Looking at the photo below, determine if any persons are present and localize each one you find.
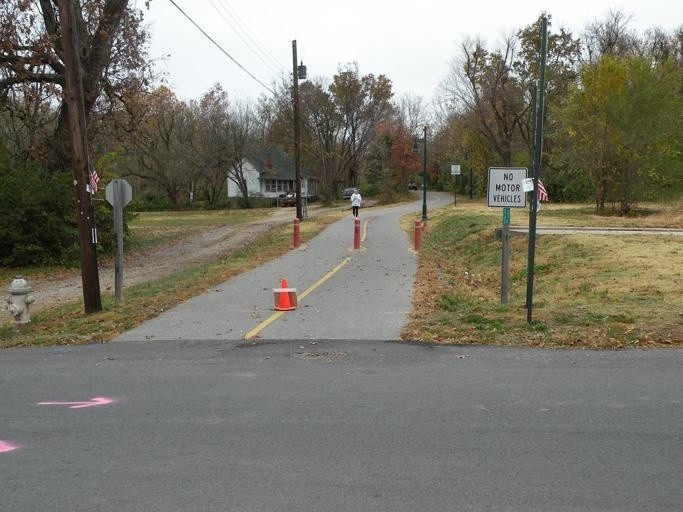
[350,189,361,219]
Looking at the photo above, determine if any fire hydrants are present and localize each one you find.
[5,274,35,329]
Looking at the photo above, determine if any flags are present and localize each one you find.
[87,157,100,194]
[537,176,549,202]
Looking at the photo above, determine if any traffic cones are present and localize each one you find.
[273,278,297,312]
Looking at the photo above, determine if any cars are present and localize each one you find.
[340,185,362,201]
[407,182,417,190]
[275,190,308,207]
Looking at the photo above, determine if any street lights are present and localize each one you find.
[290,57,307,222]
[431,160,440,192]
[410,124,430,222]
